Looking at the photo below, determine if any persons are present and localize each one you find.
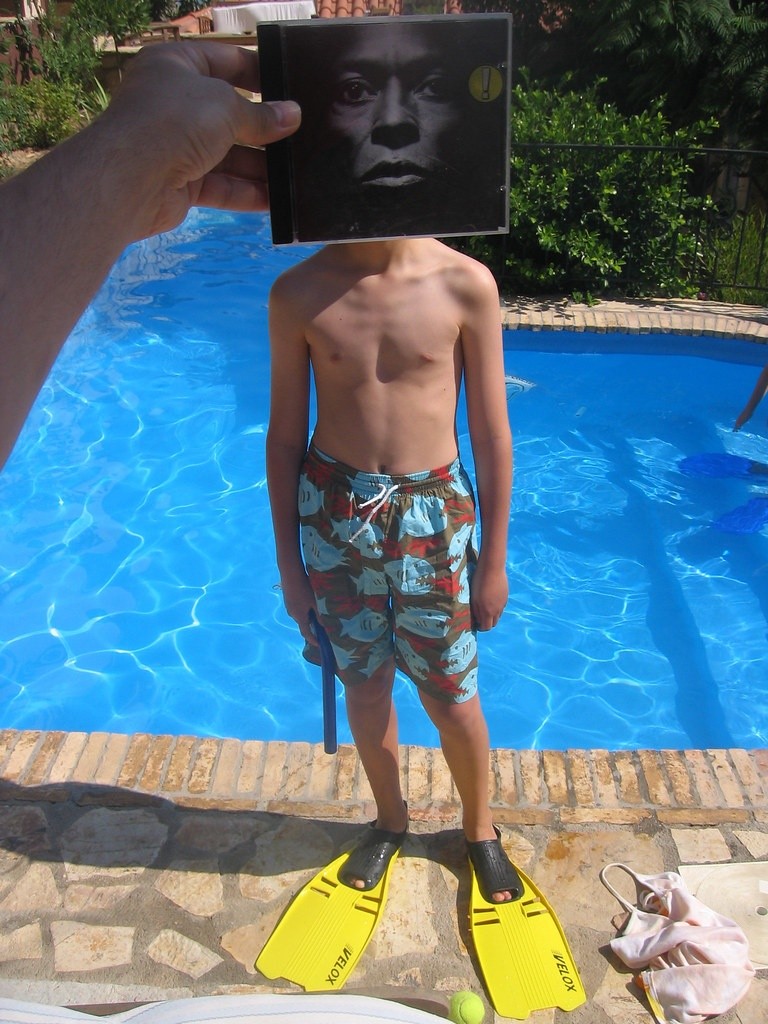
[263,236,525,905]
[309,22,501,236]
[0,33,300,471]
